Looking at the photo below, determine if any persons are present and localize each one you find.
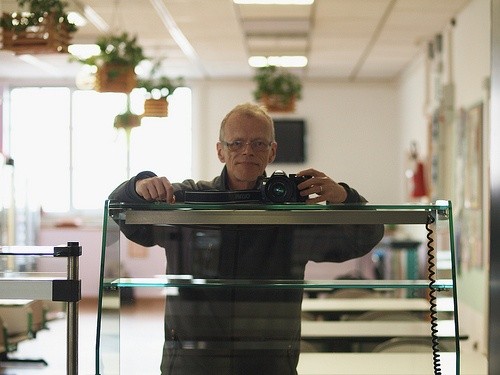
[105,103,385,375]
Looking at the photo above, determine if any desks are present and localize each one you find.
[297,279,456,375]
[0,298,48,366]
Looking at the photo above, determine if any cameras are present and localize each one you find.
[255,170,312,203]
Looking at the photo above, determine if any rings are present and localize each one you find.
[319,184,325,195]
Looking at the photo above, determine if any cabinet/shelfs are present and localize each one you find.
[95,197,461,375]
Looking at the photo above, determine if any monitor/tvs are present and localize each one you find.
[272,119,305,163]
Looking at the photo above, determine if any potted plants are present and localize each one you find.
[144,61,181,116]
[0,0,76,57]
[79,32,148,93]
[254,65,304,112]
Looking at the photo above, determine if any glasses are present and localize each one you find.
[221,139,274,150]
